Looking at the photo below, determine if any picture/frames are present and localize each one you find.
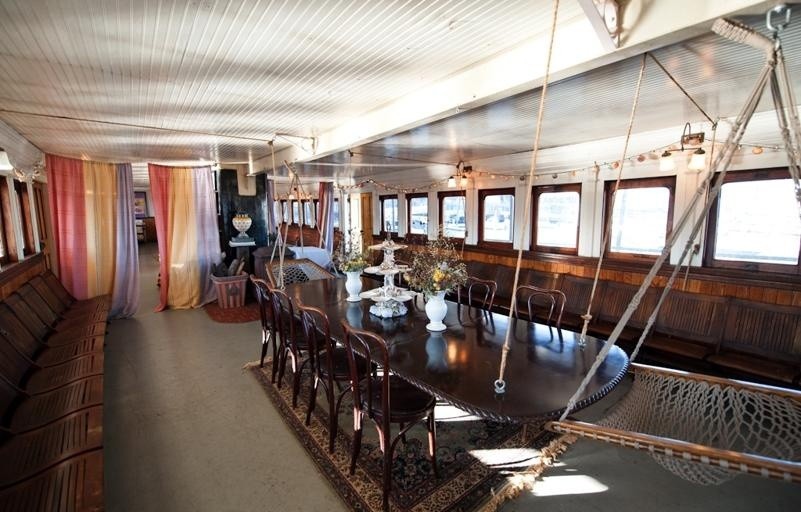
[133,191,147,219]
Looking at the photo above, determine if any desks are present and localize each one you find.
[270,275,629,445]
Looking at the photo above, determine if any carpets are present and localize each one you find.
[203,297,271,324]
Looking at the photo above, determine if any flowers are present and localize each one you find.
[333,229,370,273]
[408,230,467,293]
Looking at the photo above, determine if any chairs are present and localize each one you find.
[336,317,438,512]
[1,270,113,512]
[294,301,377,453]
[250,276,299,383]
[269,290,336,409]
[469,261,799,386]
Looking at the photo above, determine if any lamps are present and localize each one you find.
[0,145,24,182]
[448,160,468,187]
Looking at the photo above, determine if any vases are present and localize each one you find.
[231,215,251,238]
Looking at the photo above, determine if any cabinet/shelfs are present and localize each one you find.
[359,222,416,317]
